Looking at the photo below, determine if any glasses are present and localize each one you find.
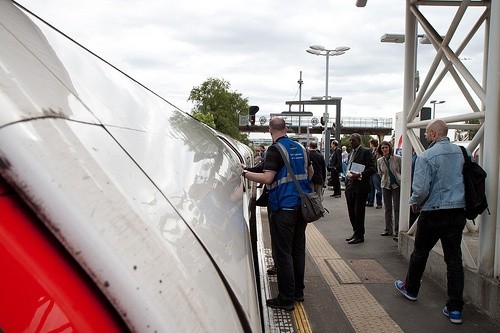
[423,129,432,136]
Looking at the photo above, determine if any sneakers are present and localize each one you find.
[394,279,417,301]
[442,304,463,324]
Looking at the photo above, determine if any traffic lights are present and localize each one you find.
[249,106,259,116]
[249,115,256,123]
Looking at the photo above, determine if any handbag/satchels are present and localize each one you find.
[298,192,325,223]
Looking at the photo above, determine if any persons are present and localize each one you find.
[409,138,421,229]
[308,141,326,202]
[254,149,261,166]
[256,145,267,188]
[394,119,475,323]
[327,140,349,198]
[242,119,314,310]
[301,141,308,153]
[339,133,401,244]
[242,142,276,276]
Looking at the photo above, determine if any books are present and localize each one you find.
[350,162,365,180]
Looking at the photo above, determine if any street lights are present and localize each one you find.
[306,44,350,118]
[430,100,446,119]
[379,33,447,101]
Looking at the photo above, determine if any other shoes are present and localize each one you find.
[267,266,277,274]
[266,296,295,311]
[394,232,398,236]
[381,231,392,236]
[330,194,341,198]
[346,231,364,244]
[276,293,304,302]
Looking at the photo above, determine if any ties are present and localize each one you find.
[349,150,355,163]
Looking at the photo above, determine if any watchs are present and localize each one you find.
[243,170,248,176]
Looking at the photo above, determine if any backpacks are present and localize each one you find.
[458,145,488,220]
